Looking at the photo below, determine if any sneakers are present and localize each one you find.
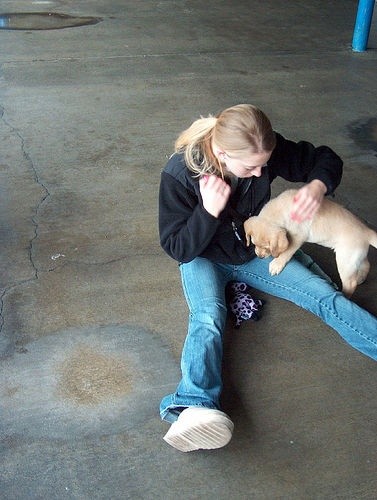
[163,405,236,454]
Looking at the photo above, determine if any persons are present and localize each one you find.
[156,102,377,453]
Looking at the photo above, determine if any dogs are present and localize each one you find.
[243,188,377,300]
